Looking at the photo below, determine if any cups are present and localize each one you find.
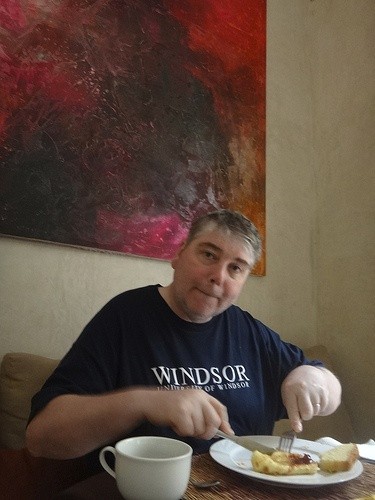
[99,436,193,500]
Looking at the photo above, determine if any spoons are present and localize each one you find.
[189,478,221,489]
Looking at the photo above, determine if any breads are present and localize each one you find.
[251,450,316,475]
[318,442,359,472]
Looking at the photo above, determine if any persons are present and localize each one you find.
[25,208,343,475]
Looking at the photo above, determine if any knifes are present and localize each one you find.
[215,429,277,456]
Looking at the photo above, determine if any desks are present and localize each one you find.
[55,443,375,500]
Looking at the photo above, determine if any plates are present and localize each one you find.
[209,436,364,489]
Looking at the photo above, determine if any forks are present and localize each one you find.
[278,415,303,454]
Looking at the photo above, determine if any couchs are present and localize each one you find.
[0,344,359,500]
